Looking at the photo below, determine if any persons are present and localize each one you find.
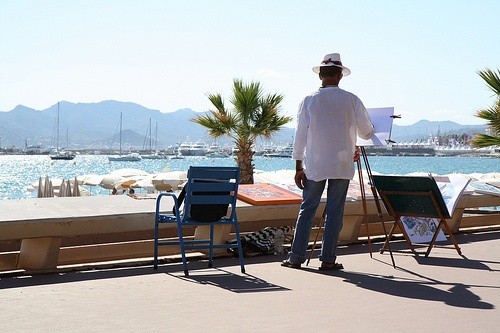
[281,52,373,271]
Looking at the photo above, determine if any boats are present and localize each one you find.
[139,116,294,160]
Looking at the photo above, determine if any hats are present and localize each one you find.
[312,53,352,78]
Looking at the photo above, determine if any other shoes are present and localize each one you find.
[281,259,301,268]
[319,263,343,271]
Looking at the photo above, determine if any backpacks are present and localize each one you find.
[173,179,230,222]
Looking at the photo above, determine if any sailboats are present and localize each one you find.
[107,111,143,162]
[22,101,77,161]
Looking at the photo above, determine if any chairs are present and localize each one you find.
[152,166,244,275]
